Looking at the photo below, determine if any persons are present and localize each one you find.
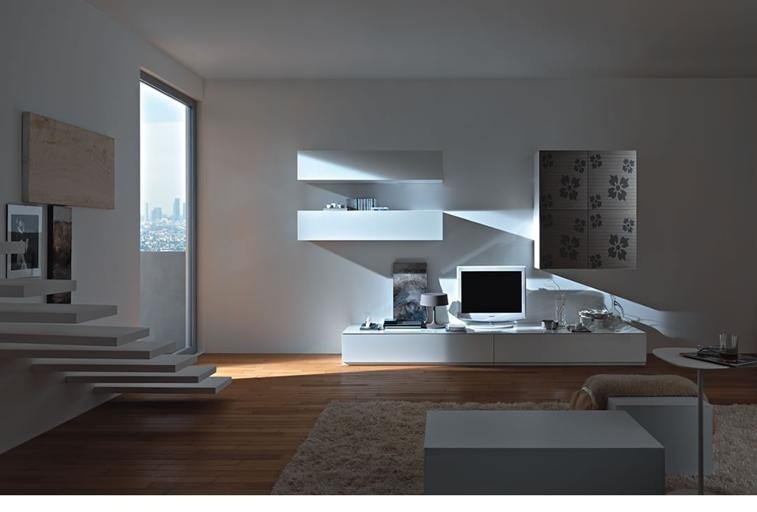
[15,215,32,269]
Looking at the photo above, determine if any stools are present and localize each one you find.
[605,396,712,476]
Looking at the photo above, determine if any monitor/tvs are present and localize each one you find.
[457,266,526,329]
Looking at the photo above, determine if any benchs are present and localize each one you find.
[424,411,666,496]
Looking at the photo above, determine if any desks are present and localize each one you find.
[652,347,757,492]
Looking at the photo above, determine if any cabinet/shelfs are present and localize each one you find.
[534,150,638,271]
[340,322,648,367]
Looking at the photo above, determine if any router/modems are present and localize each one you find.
[445,323,465,331]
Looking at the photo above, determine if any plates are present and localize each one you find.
[586,310,613,319]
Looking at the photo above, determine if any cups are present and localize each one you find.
[574,322,582,330]
[720,332,738,361]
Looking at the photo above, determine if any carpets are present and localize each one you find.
[270,399,757,495]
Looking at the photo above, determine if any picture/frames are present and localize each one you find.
[5,202,72,304]
